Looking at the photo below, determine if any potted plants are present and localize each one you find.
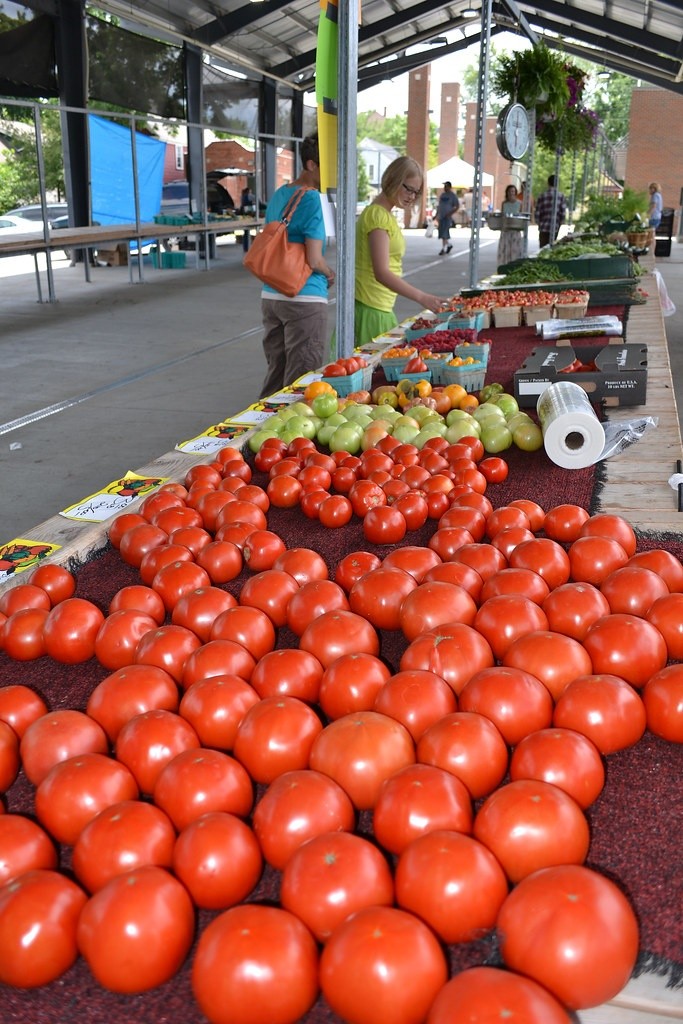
[626,226,653,249]
[498,38,571,103]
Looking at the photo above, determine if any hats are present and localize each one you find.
[443,182,451,187]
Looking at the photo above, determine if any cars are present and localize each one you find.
[0,203,71,259]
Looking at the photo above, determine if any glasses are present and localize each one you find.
[403,184,421,197]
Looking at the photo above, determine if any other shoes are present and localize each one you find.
[447,245,452,252]
[439,250,446,255]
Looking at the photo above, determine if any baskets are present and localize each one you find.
[321,309,492,400]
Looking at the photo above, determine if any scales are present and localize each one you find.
[486,71,530,229]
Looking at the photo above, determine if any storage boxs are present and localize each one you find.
[514,341,647,409]
[320,291,591,396]
[95,243,128,266]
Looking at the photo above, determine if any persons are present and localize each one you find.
[259,132,337,400]
[241,187,256,206]
[451,188,491,228]
[518,181,535,225]
[328,155,453,363]
[534,175,569,248]
[498,184,527,267]
[431,182,460,255]
[647,182,663,257]
[425,155,495,211]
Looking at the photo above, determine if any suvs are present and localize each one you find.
[164,165,253,245]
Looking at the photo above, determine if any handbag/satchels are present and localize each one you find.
[426,220,434,237]
[243,187,316,297]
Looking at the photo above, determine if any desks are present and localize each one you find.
[0,219,264,302]
[0,304,683,1024]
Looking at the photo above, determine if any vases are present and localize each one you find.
[541,110,558,123]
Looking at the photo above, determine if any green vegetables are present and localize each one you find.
[537,239,647,278]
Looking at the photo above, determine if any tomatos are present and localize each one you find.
[0,290,683,1024]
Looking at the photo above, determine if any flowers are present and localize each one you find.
[535,64,601,155]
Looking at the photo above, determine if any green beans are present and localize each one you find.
[495,260,575,286]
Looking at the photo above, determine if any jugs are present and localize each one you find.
[603,213,641,234]
